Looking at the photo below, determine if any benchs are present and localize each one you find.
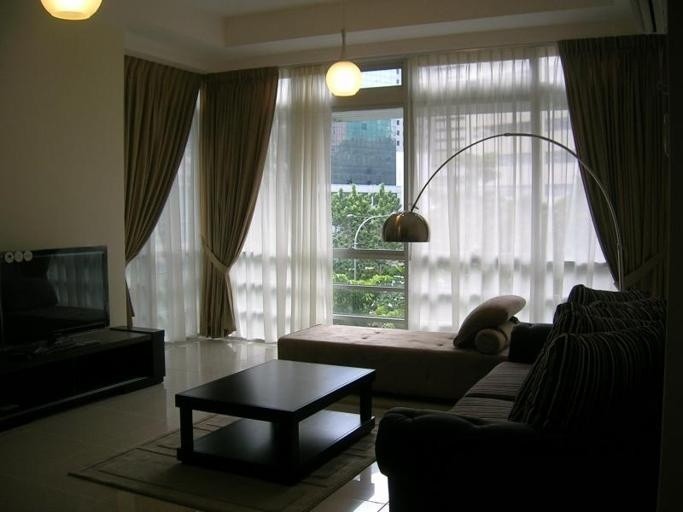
[278,321,520,400]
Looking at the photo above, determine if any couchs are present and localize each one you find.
[375,322,664,512]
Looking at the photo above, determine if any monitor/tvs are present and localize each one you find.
[0,247,109,355]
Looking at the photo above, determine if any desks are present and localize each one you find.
[0,325,165,432]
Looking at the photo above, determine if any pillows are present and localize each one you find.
[453,295,526,349]
[475,316,520,354]
[506,286,665,429]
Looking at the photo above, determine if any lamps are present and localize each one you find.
[381,133,624,292]
[325,29,361,97]
[41,0,102,20]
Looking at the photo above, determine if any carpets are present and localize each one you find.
[67,404,380,512]
